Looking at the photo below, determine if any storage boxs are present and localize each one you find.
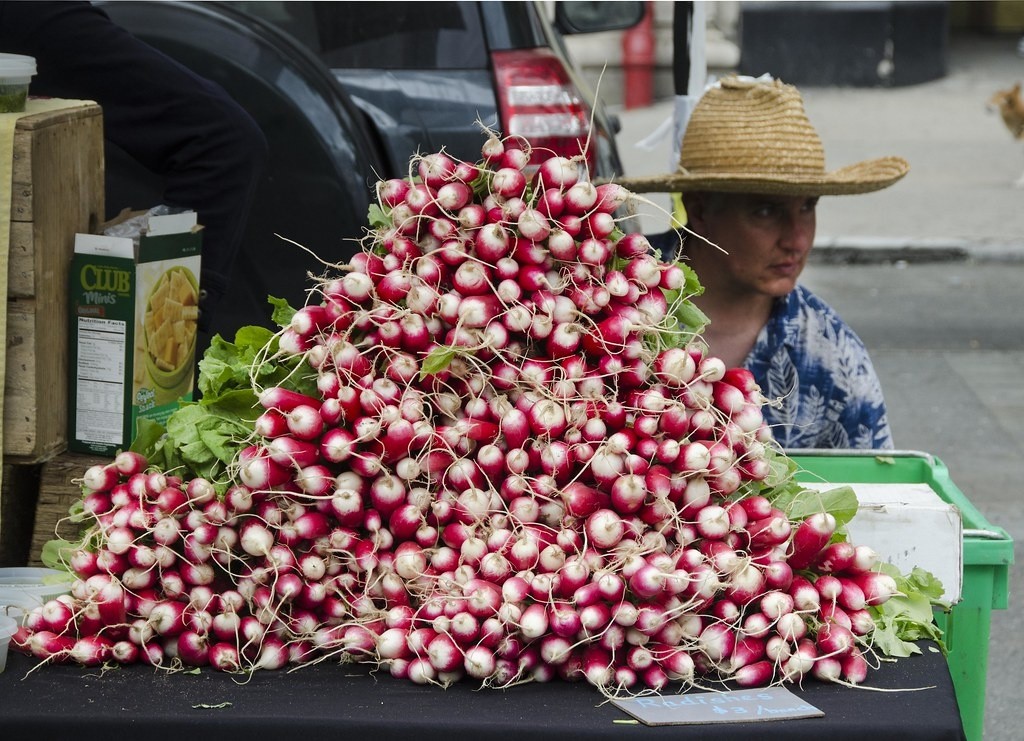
[1,97,106,466]
[798,481,965,607]
[781,449,1014,741]
[68,208,205,459]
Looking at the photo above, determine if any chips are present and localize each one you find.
[144,268,198,371]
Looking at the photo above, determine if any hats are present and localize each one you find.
[598,75,911,197]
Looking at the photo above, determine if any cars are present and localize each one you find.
[0,0,640,339]
[541,2,710,235]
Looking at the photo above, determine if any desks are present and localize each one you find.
[0,620,967,741]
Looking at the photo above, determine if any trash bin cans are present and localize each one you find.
[766,445,1015,741]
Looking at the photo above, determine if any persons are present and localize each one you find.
[643,101,895,450]
